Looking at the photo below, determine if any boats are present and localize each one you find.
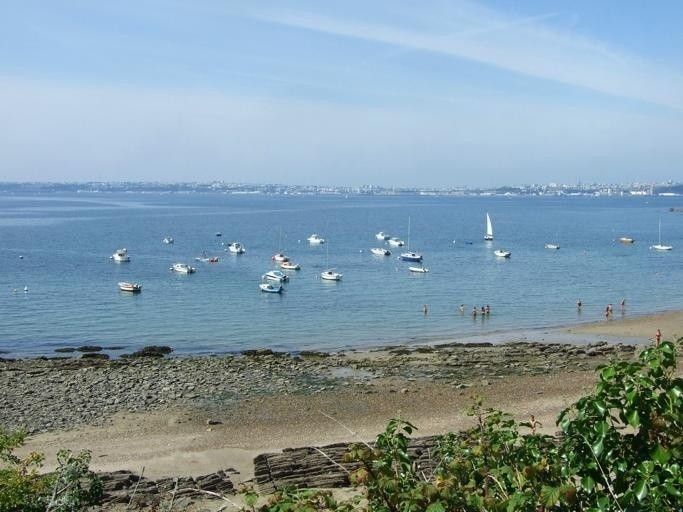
[493,249,511,257]
[110,249,131,263]
[162,232,247,273]
[650,220,673,250]
[259,233,343,293]
[371,217,430,273]
[117,281,143,291]
[544,243,560,249]
[619,237,635,244]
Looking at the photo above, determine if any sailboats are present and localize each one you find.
[482,212,494,240]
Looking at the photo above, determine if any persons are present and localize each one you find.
[470,305,477,317]
[575,296,581,312]
[605,306,610,321]
[655,327,661,346]
[608,303,612,316]
[458,303,464,313]
[480,306,485,316]
[620,296,625,312]
[485,304,490,314]
[422,304,428,314]
